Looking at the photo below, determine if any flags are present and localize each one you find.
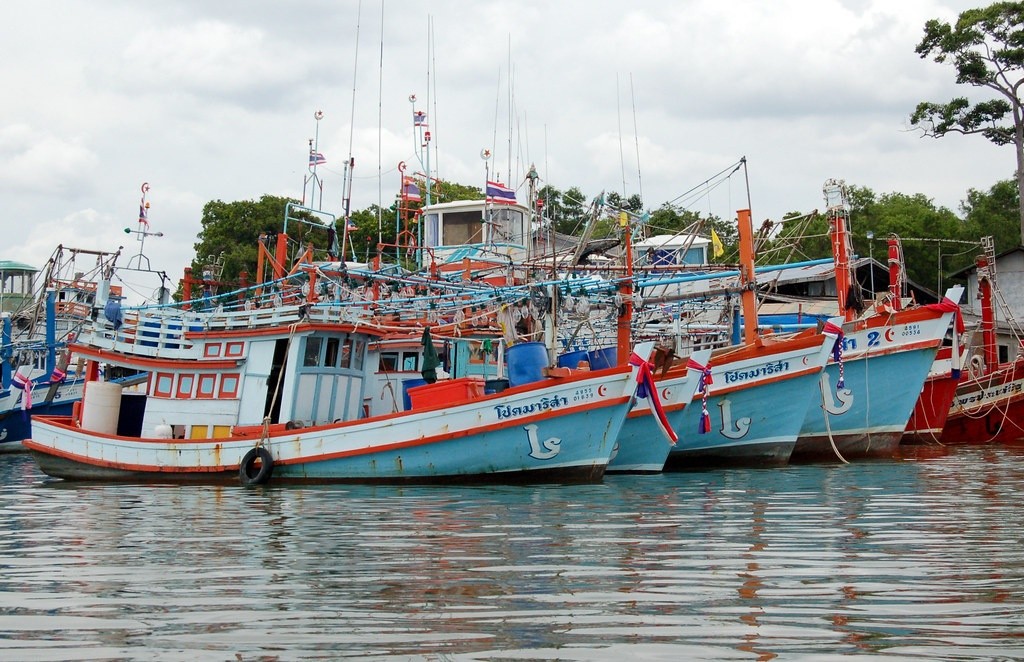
[712,229,724,259]
[485,181,518,205]
[403,176,421,198]
[138,203,149,232]
[347,218,358,231]
[309,153,326,165]
[414,111,431,127]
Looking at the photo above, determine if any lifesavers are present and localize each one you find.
[239,447,274,485]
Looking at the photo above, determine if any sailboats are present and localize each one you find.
[0,0,1023,484]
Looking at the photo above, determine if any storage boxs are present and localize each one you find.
[406,377,485,409]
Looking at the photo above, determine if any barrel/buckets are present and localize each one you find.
[558,350,588,368]
[589,347,617,370]
[82,381,122,435]
[401,378,427,410]
[506,342,550,388]
